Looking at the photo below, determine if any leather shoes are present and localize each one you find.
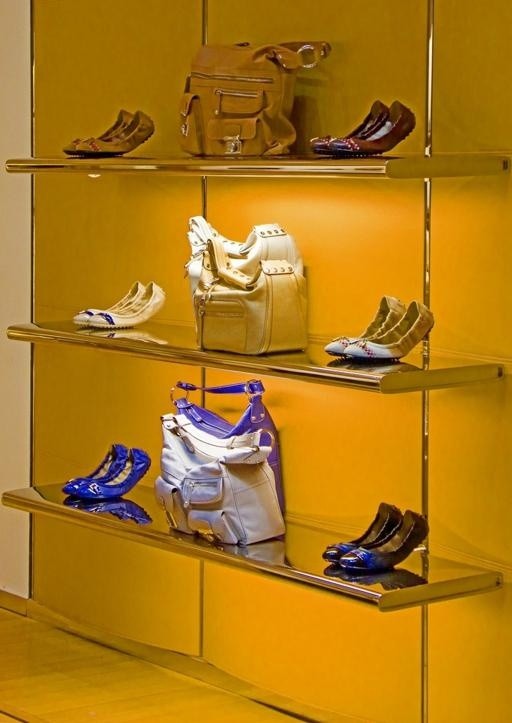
[309,100,415,153]
[63,444,150,499]
[325,564,428,589]
[324,296,434,358]
[323,503,428,570]
[63,496,152,525]
[73,281,165,329]
[63,110,154,153]
[77,330,167,344]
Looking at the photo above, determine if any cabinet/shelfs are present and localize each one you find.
[0,0,512,722]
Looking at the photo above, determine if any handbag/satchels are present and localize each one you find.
[154,379,287,545]
[184,216,307,356]
[178,42,331,157]
